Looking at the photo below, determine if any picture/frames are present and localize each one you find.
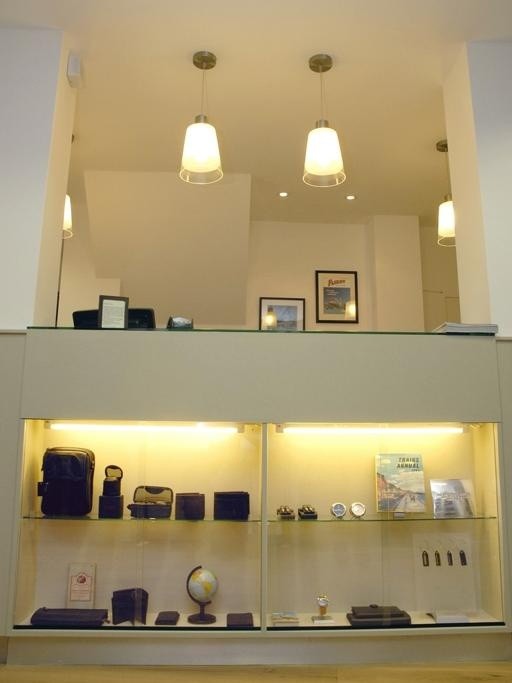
[315,269,360,324]
[98,295,130,329]
[258,297,306,331]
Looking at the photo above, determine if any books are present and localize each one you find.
[431,478,477,518]
[375,454,427,513]
[433,321,499,334]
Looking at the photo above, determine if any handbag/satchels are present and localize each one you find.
[37,447,95,516]
[347,604,411,627]
[31,608,107,626]
[128,486,173,518]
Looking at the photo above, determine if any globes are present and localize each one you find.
[185,565,218,624]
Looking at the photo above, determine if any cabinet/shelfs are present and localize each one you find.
[260,419,512,638]
[12,417,266,631]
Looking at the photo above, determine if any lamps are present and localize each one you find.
[275,424,472,438]
[60,195,75,239]
[303,53,347,188]
[435,139,458,249]
[43,421,246,436]
[179,50,225,186]
